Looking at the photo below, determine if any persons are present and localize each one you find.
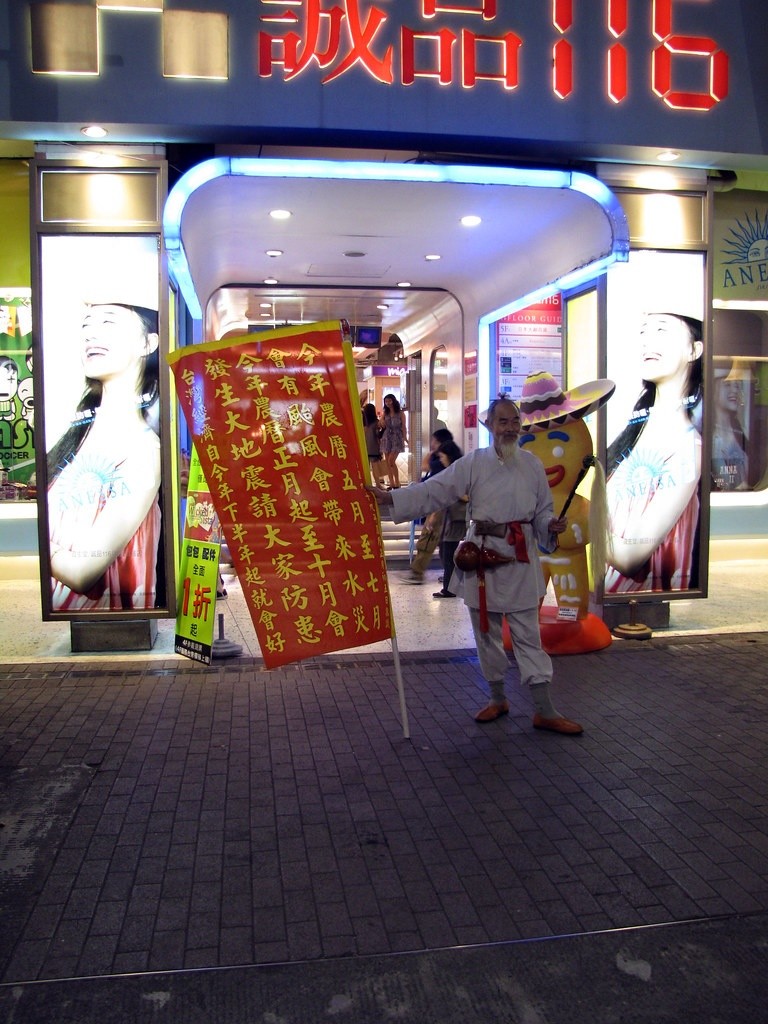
[44,293,164,610]
[365,394,585,733]
[399,429,469,597]
[711,369,750,491]
[362,394,407,490]
[606,302,701,593]
[477,369,615,620]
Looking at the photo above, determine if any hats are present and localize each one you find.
[84,254,158,312]
[647,282,705,321]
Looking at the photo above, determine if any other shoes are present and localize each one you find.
[438,577,444,583]
[433,590,456,598]
[376,484,400,491]
[474,699,510,723]
[400,570,425,584]
[534,712,584,734]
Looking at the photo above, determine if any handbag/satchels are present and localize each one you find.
[449,501,467,523]
